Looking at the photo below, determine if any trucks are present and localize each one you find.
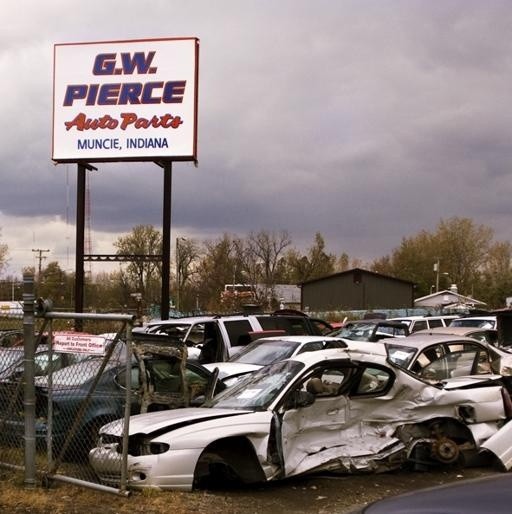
[221,282,262,309]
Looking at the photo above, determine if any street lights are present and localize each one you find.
[175,235,186,309]
[429,284,435,295]
[436,259,449,292]
